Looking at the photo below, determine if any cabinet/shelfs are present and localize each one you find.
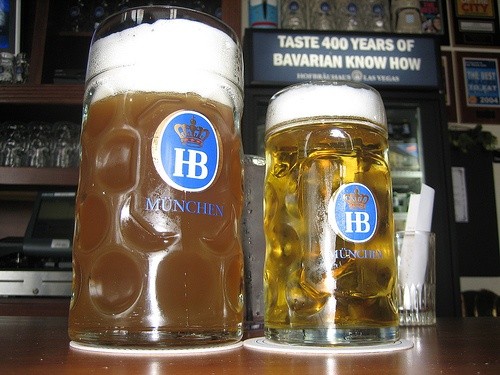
[0,0,242,183]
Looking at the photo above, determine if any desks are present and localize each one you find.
[0,297,500,375]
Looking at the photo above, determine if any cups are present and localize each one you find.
[67,4,247,347]
[0,119,81,169]
[249,0,442,32]
[262,78,401,348]
[0,51,30,86]
[393,229,438,327]
[246,156,266,329]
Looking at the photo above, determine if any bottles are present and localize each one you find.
[388,115,413,138]
[393,190,415,212]
